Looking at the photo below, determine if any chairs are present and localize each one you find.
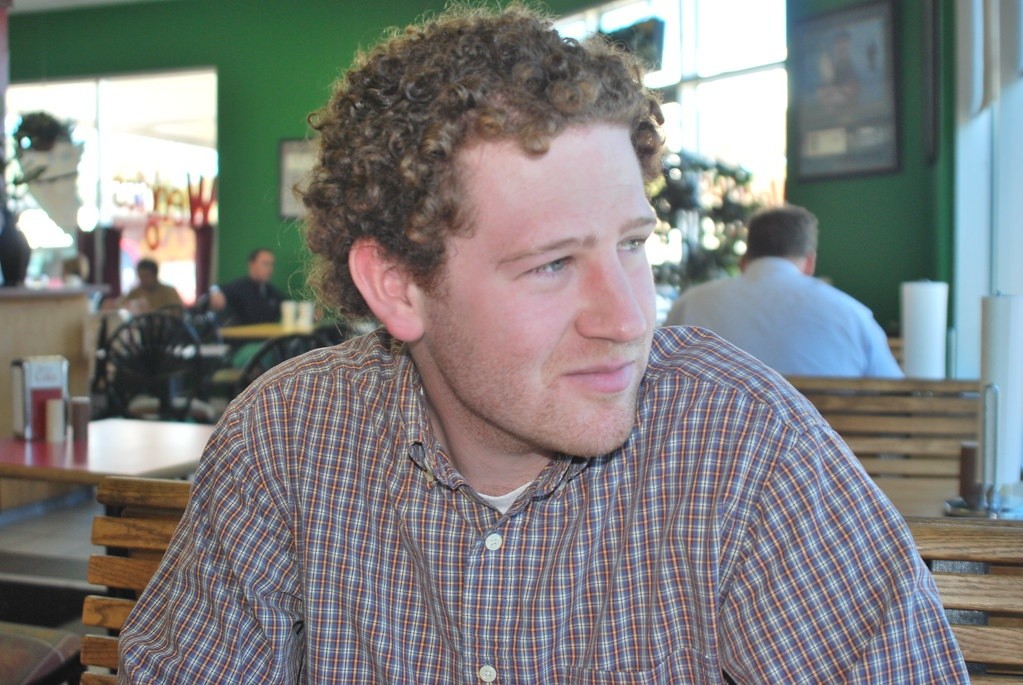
[92,294,359,426]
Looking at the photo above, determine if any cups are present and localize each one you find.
[297,302,312,328]
[281,302,296,328]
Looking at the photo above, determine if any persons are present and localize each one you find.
[206,248,300,369]
[112,258,184,418]
[115,0,970,685]
[664,206,906,378]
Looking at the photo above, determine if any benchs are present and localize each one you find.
[78,377,1023,685]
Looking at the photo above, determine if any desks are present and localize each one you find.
[218,321,317,340]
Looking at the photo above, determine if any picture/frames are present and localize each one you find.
[278,137,318,220]
[795,0,903,183]
